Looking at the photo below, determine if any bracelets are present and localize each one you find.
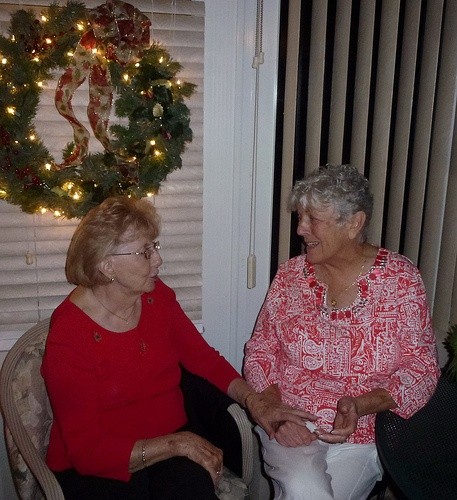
[142,439,148,468]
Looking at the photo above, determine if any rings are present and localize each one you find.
[217,471,220,475]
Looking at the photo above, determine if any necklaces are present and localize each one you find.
[91,283,137,324]
[320,241,366,306]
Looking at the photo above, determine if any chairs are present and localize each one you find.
[0,319,253,500]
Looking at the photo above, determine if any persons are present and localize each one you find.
[241,164,441,500]
[43,193,319,500]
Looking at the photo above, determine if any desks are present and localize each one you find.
[373,371,457,500]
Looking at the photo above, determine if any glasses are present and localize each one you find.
[102,239,161,258]
